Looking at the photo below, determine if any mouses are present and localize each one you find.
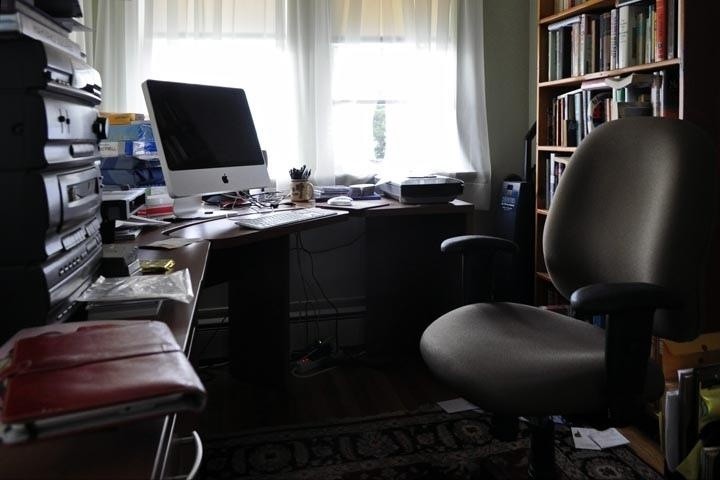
[326,195,353,206]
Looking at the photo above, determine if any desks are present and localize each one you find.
[0,189,476,480]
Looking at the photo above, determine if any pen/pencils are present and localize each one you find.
[289,165,311,179]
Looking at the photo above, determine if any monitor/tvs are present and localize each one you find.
[141,79,273,222]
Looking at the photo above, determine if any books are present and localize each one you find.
[545,152,574,210]
[546,67,678,147]
[544,1,678,81]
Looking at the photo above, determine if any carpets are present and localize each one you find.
[198,407,666,480]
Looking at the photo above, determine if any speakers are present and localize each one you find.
[494,179,527,290]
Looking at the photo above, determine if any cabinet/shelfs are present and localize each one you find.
[535,0,720,480]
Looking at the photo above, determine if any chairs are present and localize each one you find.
[419,115,717,480]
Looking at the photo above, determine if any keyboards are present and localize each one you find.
[235,207,337,231]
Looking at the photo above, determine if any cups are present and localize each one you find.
[289,178,315,202]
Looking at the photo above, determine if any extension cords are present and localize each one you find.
[296,345,338,374]
